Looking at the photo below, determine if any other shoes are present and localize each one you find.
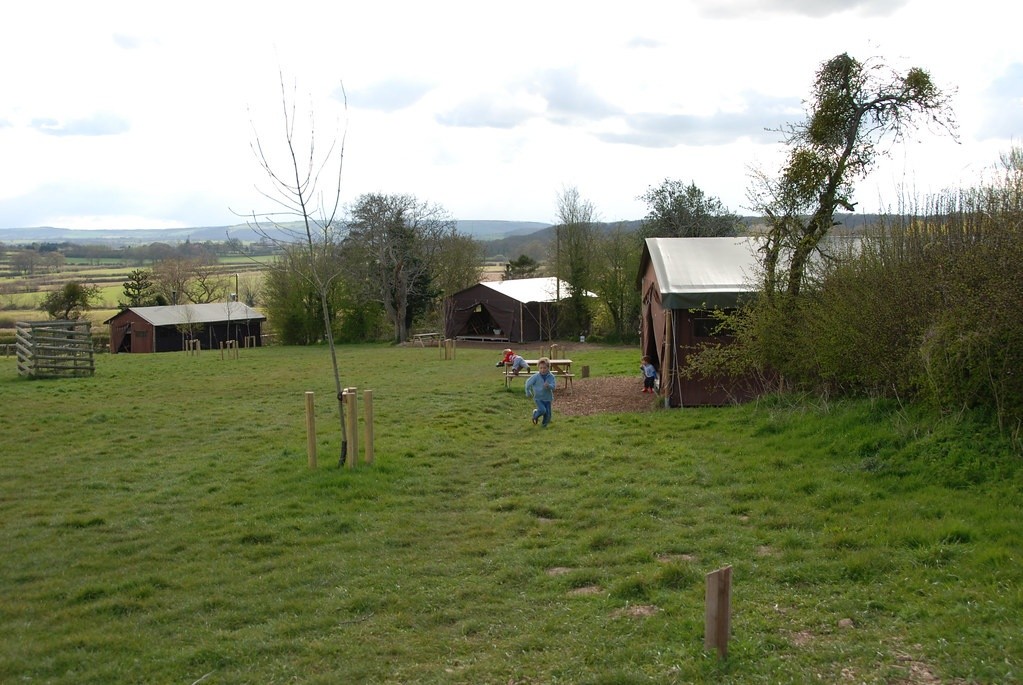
[641,387,648,392]
[513,370,518,375]
[528,367,530,374]
[648,387,652,393]
[533,409,538,424]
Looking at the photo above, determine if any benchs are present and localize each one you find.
[502,371,575,378]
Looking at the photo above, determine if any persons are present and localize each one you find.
[639,355,657,394]
[498,349,531,376]
[525,357,556,429]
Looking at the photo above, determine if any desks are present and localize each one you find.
[411,332,440,348]
[506,359,575,393]
